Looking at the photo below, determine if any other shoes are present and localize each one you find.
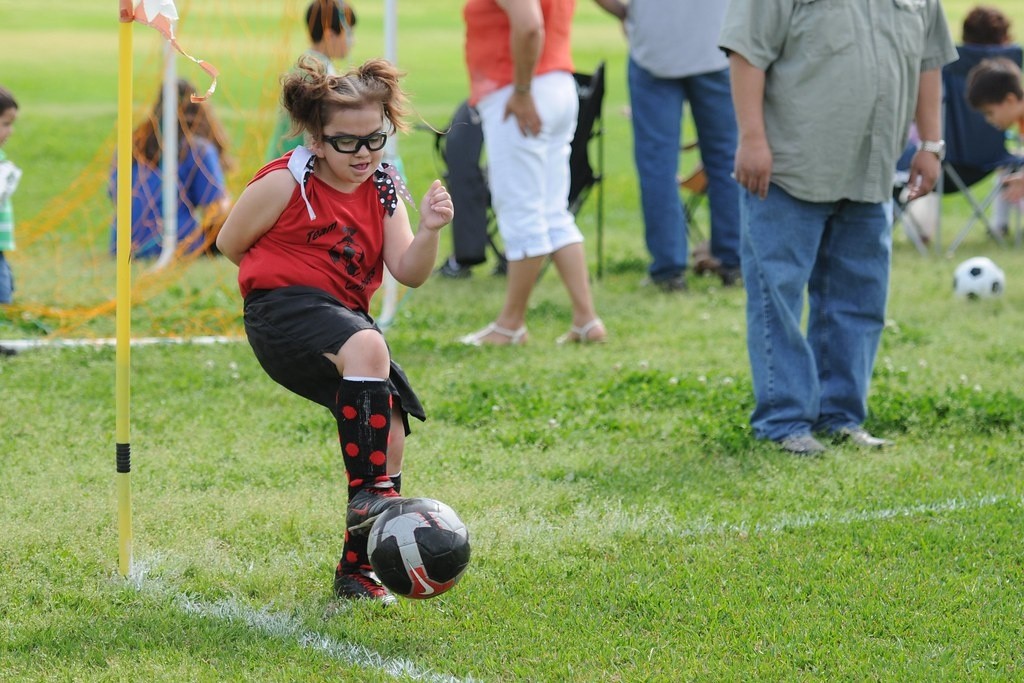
[719,266,744,289]
[488,258,508,277]
[638,273,689,298]
[431,259,471,280]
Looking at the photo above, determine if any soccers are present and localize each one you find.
[952,257,1009,302]
[366,496,472,600]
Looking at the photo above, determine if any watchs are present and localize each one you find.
[917,140,947,160]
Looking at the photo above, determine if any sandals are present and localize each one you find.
[461,323,527,349]
[556,319,606,347]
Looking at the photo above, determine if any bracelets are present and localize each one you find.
[514,85,529,91]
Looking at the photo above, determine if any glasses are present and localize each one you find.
[312,130,387,153]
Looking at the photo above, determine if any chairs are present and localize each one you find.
[886,43,1024,261]
[432,63,608,279]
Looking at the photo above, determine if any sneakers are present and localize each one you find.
[828,425,893,452]
[334,565,398,610]
[344,480,406,537]
[776,435,824,457]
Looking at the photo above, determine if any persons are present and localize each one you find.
[0,87,19,356]
[215,62,454,606]
[109,80,224,253]
[898,6,1024,243]
[597,1,742,289]
[436,1,605,347]
[718,0,959,452]
[266,0,355,162]
